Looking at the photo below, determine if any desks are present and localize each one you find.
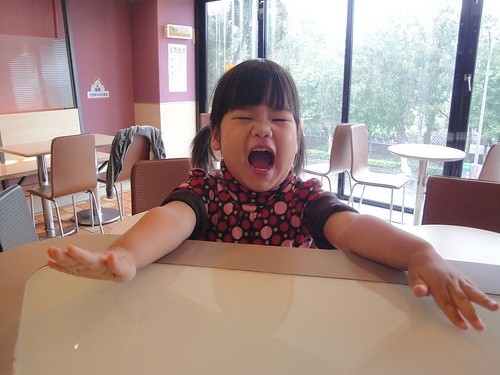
[0,133,122,240]
[0,234,500,375]
[388,143,466,225]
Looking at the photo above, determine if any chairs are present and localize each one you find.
[130,158,201,216]
[293,123,354,209]
[89,125,166,227]
[346,124,411,225]
[26,133,105,237]
[421,142,500,233]
[200,113,223,169]
[0,184,39,253]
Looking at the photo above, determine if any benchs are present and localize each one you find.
[0,151,111,190]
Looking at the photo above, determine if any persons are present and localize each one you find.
[46,57,500,330]
